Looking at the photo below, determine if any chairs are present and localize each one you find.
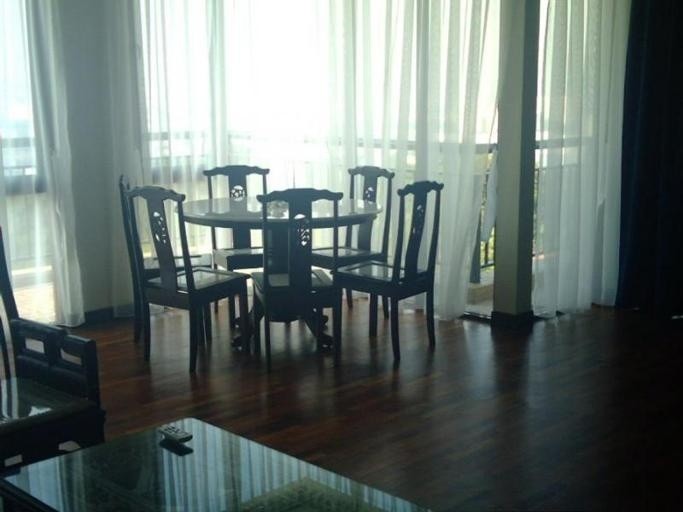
[310,166,395,318]
[329,179,444,365]
[1,312,108,475]
[205,165,269,312]
[114,174,212,348]
[252,188,343,372]
[124,186,251,373]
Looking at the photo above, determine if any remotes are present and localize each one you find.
[159,426,193,442]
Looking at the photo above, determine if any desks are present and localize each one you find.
[174,193,384,351]
